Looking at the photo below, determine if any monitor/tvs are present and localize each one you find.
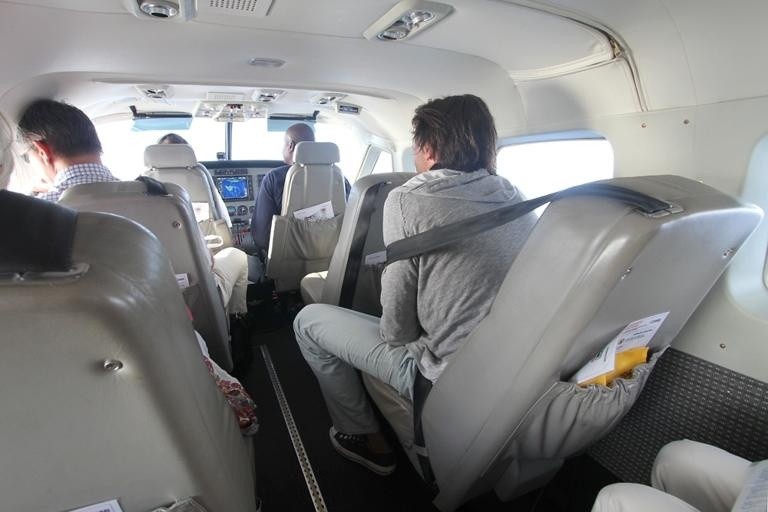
[213,175,254,201]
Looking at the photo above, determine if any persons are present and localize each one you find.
[0,109,264,436]
[291,88,546,479]
[160,129,237,231]
[15,96,257,333]
[242,122,351,281]
[592,432,767,510]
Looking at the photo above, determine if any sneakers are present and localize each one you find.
[329,425,400,477]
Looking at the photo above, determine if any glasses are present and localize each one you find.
[20,147,33,163]
